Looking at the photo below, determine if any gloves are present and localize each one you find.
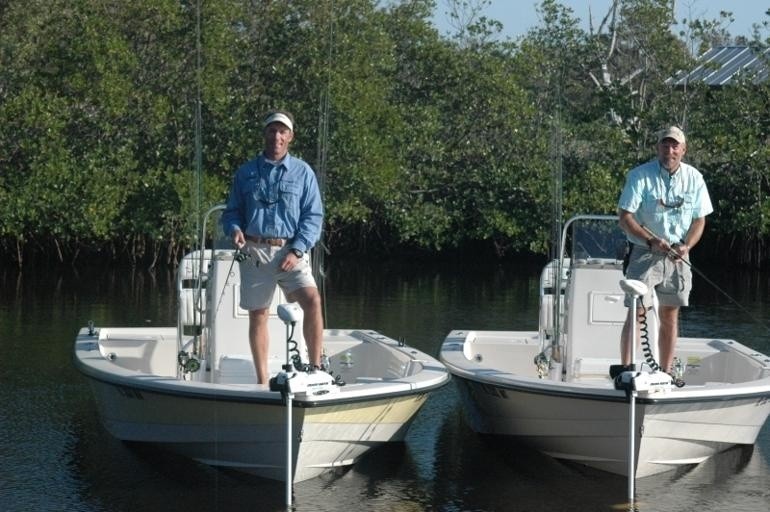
[647,235,670,252]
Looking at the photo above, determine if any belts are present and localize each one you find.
[244,235,283,246]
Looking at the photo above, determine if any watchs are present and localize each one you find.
[292,248,304,258]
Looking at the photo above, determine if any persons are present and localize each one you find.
[614,125,716,388]
[219,107,327,388]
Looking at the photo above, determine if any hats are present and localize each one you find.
[657,127,685,144]
[263,111,294,133]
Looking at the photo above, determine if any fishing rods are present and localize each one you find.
[208,240,245,338]
[640,223,770,332]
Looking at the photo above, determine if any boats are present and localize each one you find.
[437,71,770,503]
[69,1,455,491]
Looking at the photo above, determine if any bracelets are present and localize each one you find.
[646,236,653,246]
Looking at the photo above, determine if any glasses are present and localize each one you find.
[258,183,284,206]
[660,182,685,208]
